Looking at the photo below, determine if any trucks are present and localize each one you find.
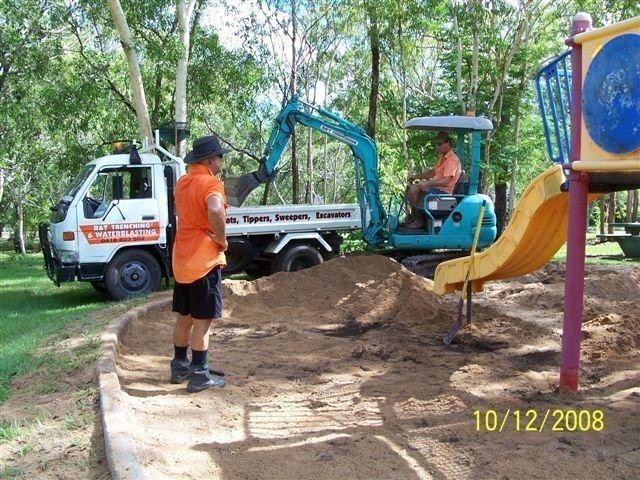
[38,129,370,301]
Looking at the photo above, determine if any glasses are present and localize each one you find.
[218,153,224,159]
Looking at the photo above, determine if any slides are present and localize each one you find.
[434,163,604,295]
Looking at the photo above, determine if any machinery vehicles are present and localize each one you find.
[223,93,498,278]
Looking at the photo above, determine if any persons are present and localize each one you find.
[169,135,229,394]
[397,131,462,230]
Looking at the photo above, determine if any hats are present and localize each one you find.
[182,136,231,163]
[430,132,451,143]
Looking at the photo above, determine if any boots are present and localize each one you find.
[185,367,226,392]
[169,360,191,383]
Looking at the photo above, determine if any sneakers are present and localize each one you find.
[406,219,425,229]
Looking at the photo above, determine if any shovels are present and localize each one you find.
[442,200,486,346]
